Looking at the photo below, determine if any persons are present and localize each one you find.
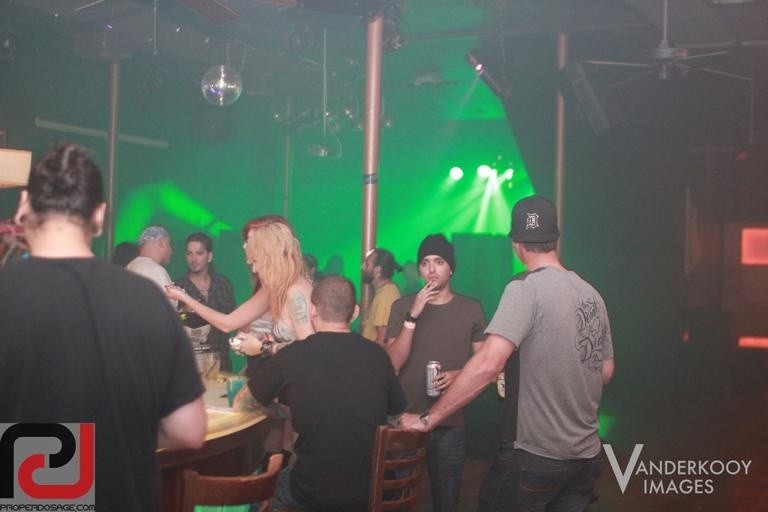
[1,137,210,511]
[227,272,408,511]
[122,223,180,315]
[379,233,491,512]
[174,233,238,373]
[357,247,407,353]
[162,216,294,375]
[399,194,615,511]
[226,223,316,407]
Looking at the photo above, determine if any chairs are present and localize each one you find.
[371,424,430,511]
[180,453,283,512]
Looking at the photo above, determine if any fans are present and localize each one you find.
[68,0,302,28]
[575,1,756,98]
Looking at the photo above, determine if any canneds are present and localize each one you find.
[426,360,442,398]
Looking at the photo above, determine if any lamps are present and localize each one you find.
[199,1,405,160]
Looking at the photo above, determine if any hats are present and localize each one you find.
[417,234,454,274]
[509,194,562,244]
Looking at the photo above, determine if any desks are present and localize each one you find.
[145,371,275,475]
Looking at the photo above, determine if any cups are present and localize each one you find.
[202,347,220,380]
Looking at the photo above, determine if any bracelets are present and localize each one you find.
[256,332,269,343]
[260,341,273,358]
[420,411,431,429]
[406,312,416,322]
[403,319,416,329]
